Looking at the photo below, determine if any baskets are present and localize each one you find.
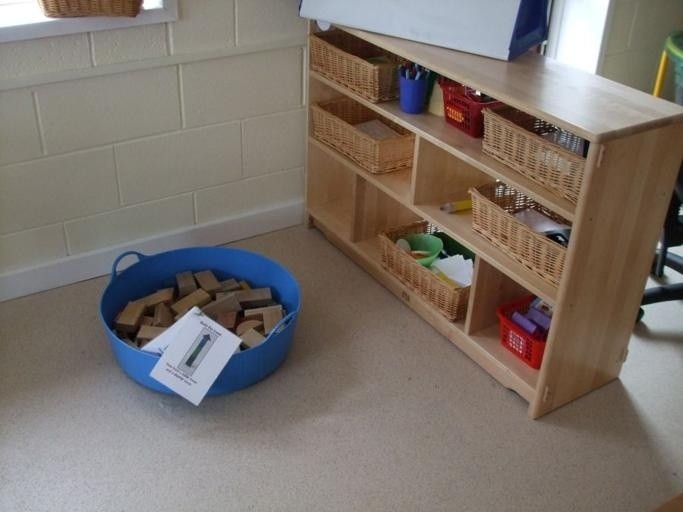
[311,96,415,173]
[470,182,572,288]
[38,0,143,17]
[481,106,587,207]
[497,295,546,370]
[309,30,415,103]
[379,221,471,321]
[440,76,536,137]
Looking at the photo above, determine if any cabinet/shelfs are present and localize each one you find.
[298,15,681,422]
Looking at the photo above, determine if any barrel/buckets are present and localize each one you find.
[398,74,430,113]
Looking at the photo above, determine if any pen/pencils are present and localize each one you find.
[440,199,473,213]
[398,60,430,80]
